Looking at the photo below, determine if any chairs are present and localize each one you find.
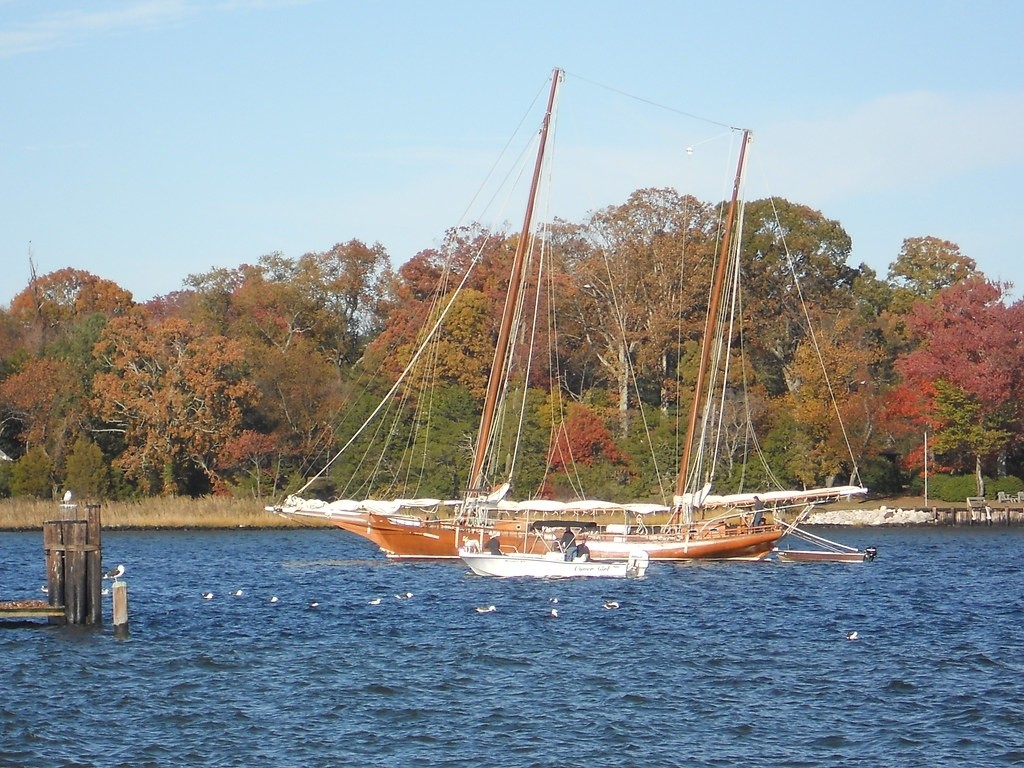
[998,491,1024,503]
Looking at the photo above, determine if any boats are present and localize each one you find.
[773,550,866,563]
[458,520,649,580]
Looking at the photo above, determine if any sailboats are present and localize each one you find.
[261,67,868,579]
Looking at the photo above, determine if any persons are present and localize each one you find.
[485,535,509,556]
[753,496,763,533]
[552,538,563,552]
[576,543,590,562]
[557,527,577,561]
[460,515,468,530]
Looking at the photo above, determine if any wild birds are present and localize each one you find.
[846,630,859,640]
[199,592,213,599]
[228,589,242,596]
[550,597,559,618]
[394,592,413,599]
[601,599,619,610]
[41,585,48,592]
[475,605,496,613]
[101,563,125,596]
[60,490,71,505]
[268,596,278,603]
[305,601,318,607]
[368,598,382,605]
